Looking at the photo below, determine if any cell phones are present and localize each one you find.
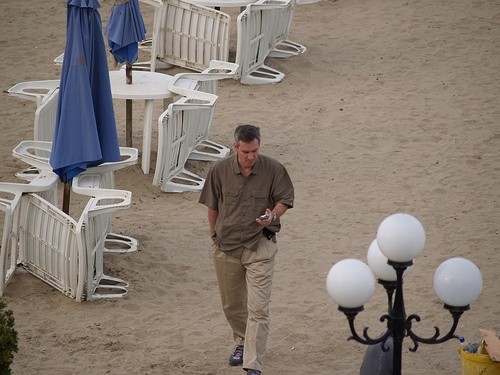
[259,215,269,221]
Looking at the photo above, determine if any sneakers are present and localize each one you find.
[228,344,244,366]
[247,369,262,375]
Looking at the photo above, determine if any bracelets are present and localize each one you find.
[272,210,277,222]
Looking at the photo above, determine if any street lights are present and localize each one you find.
[325,214,483,375]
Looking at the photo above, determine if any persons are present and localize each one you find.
[198,124,293,375]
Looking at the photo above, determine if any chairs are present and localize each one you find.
[0,0,322,304]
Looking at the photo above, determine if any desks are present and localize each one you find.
[109,68,176,147]
[187,0,258,11]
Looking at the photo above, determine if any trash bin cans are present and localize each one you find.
[457,328,500,375]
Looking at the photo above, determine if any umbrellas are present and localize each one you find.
[104,0,148,146]
[49,0,122,215]
